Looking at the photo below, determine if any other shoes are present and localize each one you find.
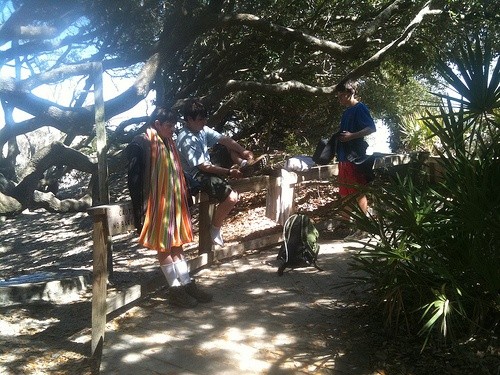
[240,153,270,176]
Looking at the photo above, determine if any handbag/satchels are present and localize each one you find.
[312,138,336,165]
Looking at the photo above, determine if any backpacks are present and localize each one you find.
[278,214,320,274]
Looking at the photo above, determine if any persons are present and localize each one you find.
[175,105,271,247]
[124,107,213,307]
[334,81,376,238]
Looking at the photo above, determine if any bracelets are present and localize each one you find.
[227,169,232,176]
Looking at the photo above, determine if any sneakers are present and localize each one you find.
[168,286,198,308]
[344,227,363,242]
[181,280,212,302]
[338,221,349,237]
